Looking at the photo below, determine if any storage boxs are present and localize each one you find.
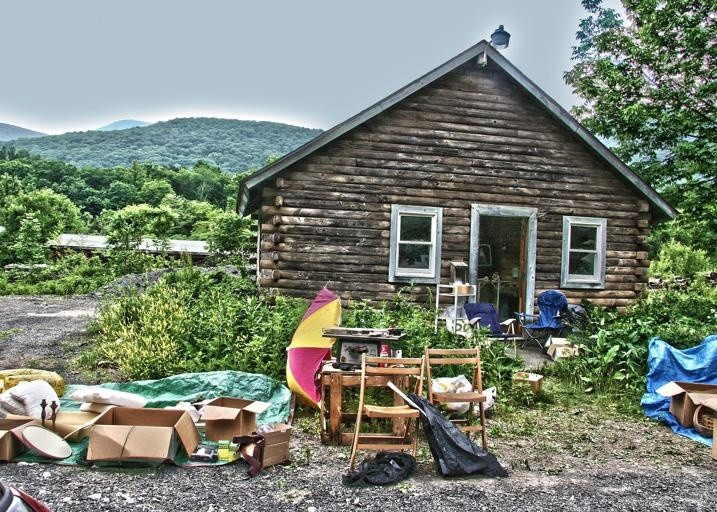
[190,395,272,444]
[655,380,717,429]
[61,406,201,465]
[513,372,543,393]
[0,417,42,462]
[544,336,572,348]
[256,422,292,469]
[701,397,717,461]
[547,344,577,362]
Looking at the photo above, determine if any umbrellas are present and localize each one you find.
[286,280,341,411]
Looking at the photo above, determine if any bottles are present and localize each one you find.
[380,345,388,367]
[391,348,402,359]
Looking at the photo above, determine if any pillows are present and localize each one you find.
[1,388,27,417]
[0,406,13,419]
[68,387,145,408]
[10,378,59,418]
[80,402,113,414]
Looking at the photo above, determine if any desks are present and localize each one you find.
[316,357,410,445]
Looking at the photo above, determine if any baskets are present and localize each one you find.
[693,405,714,438]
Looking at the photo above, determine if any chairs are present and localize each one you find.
[424,346,488,449]
[348,352,426,469]
[464,300,517,358]
[513,290,568,358]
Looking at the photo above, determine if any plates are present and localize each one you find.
[23,425,72,459]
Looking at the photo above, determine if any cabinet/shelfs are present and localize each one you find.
[435,284,478,335]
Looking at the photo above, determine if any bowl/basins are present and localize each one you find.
[458,286,472,294]
